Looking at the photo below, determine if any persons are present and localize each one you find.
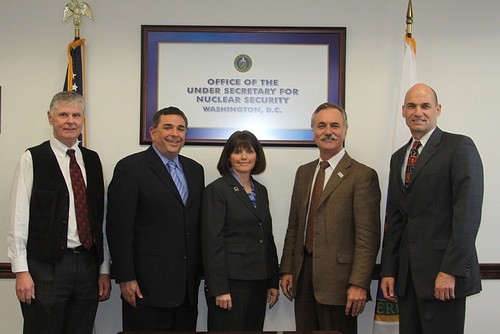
[380,83,483,334]
[201,130,279,334]
[277,103,382,334]
[107,107,205,334]
[9,91,111,334]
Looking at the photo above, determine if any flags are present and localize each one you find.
[373,36,420,334]
[64,36,88,147]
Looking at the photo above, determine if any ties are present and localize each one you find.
[66,148,93,251]
[168,161,188,206]
[404,140,422,188]
[305,160,330,255]
